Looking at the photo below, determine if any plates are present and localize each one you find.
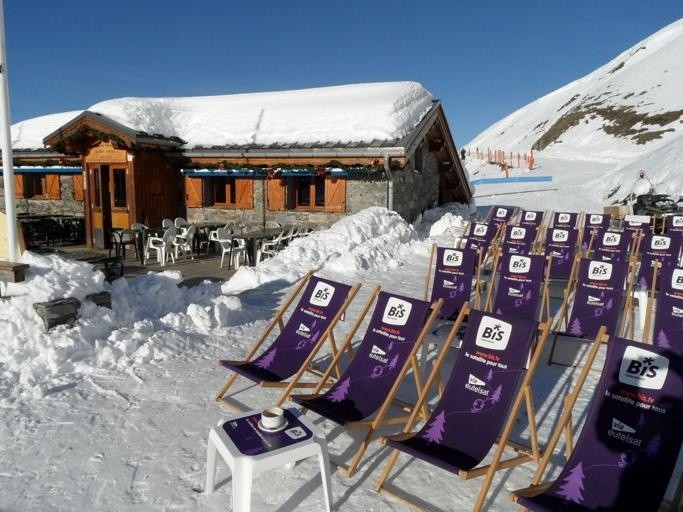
[257,417,289,432]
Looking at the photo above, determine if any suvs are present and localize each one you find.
[626,190,682,236]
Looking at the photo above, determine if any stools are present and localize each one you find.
[205,407,333,512]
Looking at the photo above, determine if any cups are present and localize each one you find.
[261,408,284,428]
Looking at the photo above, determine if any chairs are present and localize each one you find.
[106,217,309,282]
